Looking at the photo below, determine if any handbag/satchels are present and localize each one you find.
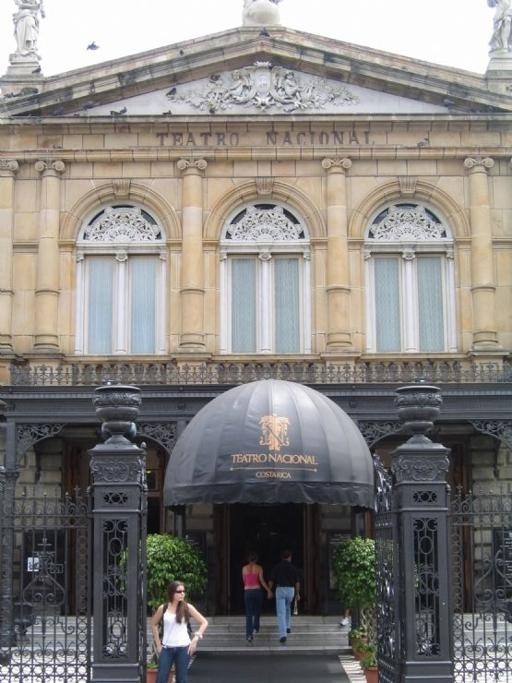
[292,594,301,616]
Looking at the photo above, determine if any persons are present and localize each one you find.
[338,606,351,627]
[149,579,209,683]
[240,550,274,643]
[266,549,302,644]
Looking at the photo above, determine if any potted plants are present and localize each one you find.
[118,533,208,683]
[332,534,417,681]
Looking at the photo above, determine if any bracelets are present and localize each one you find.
[190,631,204,640]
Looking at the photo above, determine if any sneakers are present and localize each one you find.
[340,617,349,626]
[278,626,292,644]
[246,636,254,647]
